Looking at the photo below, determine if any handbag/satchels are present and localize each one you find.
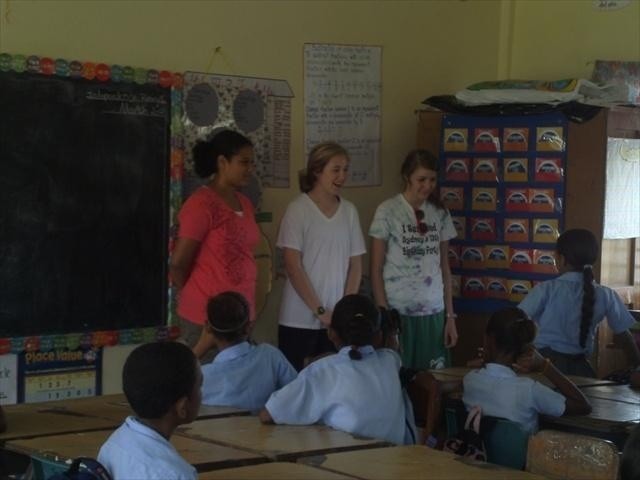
[443,406,487,462]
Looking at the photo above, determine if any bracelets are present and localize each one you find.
[445,313,457,319]
[537,357,552,376]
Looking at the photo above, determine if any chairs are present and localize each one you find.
[482,417,525,469]
[526,431,619,480]
[30,450,111,480]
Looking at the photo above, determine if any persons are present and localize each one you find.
[275,141,365,363]
[193,290,299,417]
[96,342,203,480]
[462,308,593,436]
[370,148,457,447]
[169,130,262,363]
[517,228,640,376]
[381,307,444,446]
[259,294,408,453]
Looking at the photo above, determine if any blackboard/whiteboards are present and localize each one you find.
[0,55,185,354]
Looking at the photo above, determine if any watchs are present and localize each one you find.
[313,306,328,316]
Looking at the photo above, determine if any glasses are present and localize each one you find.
[415,207,427,234]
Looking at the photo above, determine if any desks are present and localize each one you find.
[6,429,264,471]
[297,444,544,480]
[198,462,358,480]
[540,385,640,451]
[518,371,617,390]
[174,415,390,461]
[416,366,478,445]
[38,394,251,422]
[0,402,122,440]
